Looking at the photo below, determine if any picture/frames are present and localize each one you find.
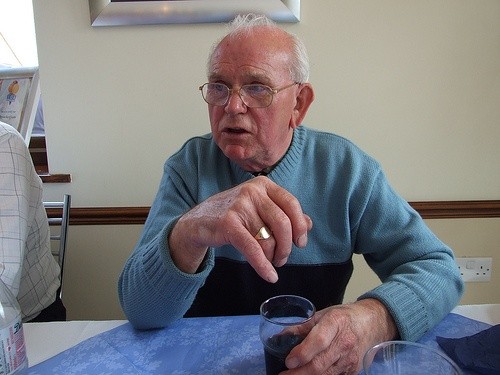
[0,65,41,149]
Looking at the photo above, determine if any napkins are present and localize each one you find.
[436,324,500,375]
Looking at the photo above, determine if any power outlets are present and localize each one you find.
[454,257,492,282]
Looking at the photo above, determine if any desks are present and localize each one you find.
[19,304,500,375]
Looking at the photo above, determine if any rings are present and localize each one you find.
[254,226,272,239]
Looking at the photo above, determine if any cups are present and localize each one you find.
[363,340,465,375]
[259,295,316,375]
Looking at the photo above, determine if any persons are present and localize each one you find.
[0,121,67,323]
[117,13,464,375]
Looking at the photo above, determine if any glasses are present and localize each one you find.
[199,81,301,108]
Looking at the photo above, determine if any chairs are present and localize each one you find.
[43,195,71,300]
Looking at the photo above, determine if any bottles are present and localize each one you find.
[0,279,29,375]
